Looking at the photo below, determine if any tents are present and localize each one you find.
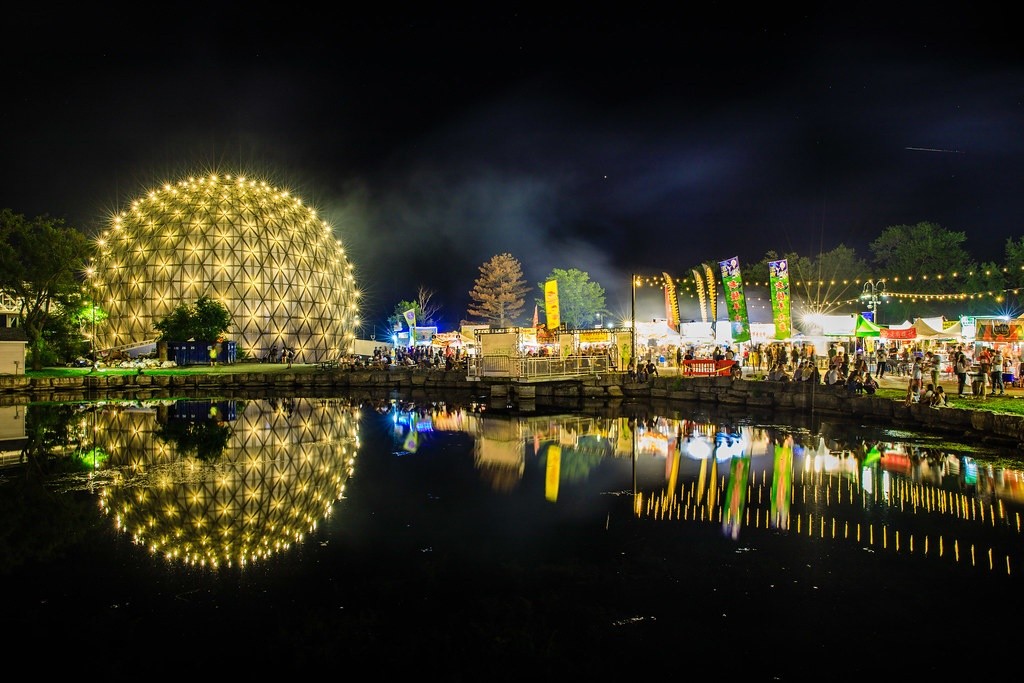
[824,314,886,362]
[895,317,962,357]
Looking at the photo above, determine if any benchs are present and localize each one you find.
[313,358,471,373]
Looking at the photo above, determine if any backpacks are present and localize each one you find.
[942,394,950,405]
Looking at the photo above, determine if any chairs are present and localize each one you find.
[1002,373,1015,388]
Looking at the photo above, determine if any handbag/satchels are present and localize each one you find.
[874,379,880,388]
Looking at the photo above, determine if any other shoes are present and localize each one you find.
[998,391,1005,395]
[959,394,966,398]
[989,391,995,395]
[901,403,913,409]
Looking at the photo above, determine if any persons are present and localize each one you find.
[888,343,901,376]
[209,346,217,367]
[676,345,695,375]
[628,358,658,381]
[901,347,911,377]
[743,342,820,383]
[340,345,460,372]
[713,346,734,362]
[269,346,294,369]
[824,345,879,395]
[526,345,616,372]
[875,342,888,379]
[908,345,1004,409]
